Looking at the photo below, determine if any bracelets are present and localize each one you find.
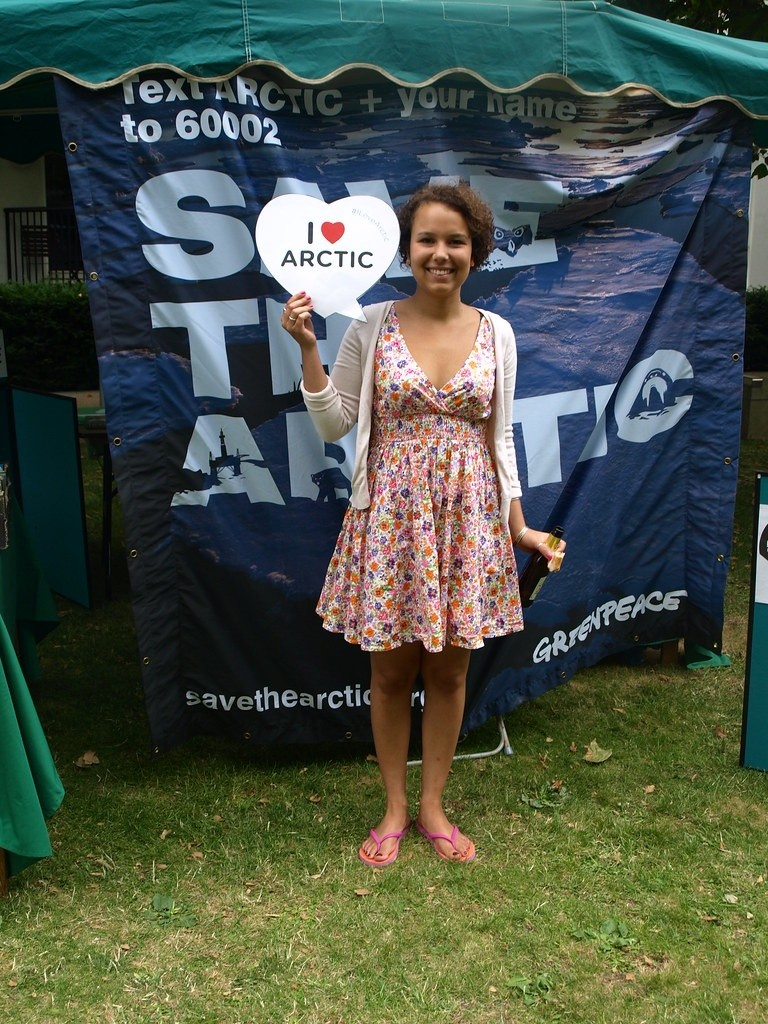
[514,527,529,545]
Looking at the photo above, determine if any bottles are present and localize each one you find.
[517,526,566,609]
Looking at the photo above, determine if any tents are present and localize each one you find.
[0,0,768,877]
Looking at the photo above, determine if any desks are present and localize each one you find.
[76,423,130,601]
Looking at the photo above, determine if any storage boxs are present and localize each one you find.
[741,371,768,441]
[53,391,100,423]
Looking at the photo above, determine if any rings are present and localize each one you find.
[536,543,543,548]
[283,308,286,311]
[288,316,296,321]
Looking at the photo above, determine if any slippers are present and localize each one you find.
[416,817,475,864]
[359,817,414,867]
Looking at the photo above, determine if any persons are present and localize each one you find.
[281,181,567,867]
